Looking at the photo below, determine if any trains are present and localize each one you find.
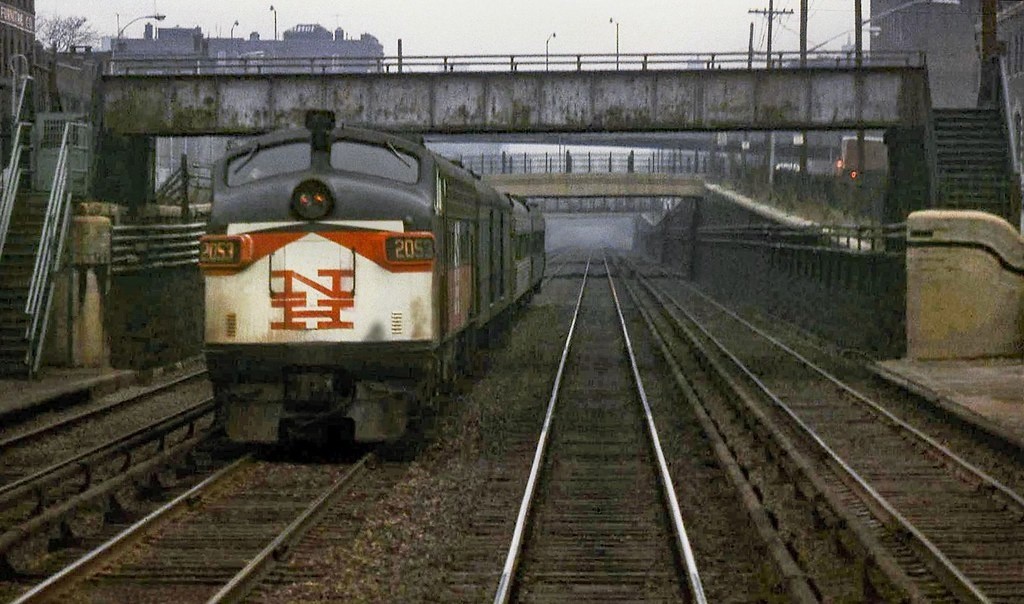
[197,124,548,452]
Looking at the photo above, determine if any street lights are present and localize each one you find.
[115,15,168,54]
[230,20,239,58]
[270,6,277,41]
[609,18,620,72]
[544,33,557,71]
